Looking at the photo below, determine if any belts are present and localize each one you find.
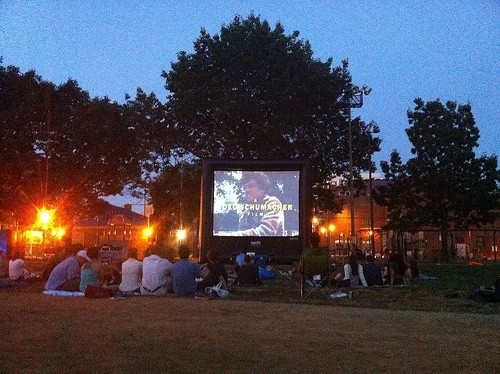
[143,285,162,293]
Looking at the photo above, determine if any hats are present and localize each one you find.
[78,249,91,263]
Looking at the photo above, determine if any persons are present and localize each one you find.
[259,264,273,280]
[140,244,173,296]
[80,246,104,293]
[171,246,202,297]
[200,249,229,291]
[232,247,260,286]
[327,250,412,288]
[0,249,29,281]
[278,230,321,279]
[45,250,89,293]
[219,171,284,237]
[118,247,143,295]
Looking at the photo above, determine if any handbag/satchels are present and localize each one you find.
[204,281,229,300]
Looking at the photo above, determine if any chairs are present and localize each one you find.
[292,247,332,301]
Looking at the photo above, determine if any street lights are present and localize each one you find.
[345,82,373,253]
